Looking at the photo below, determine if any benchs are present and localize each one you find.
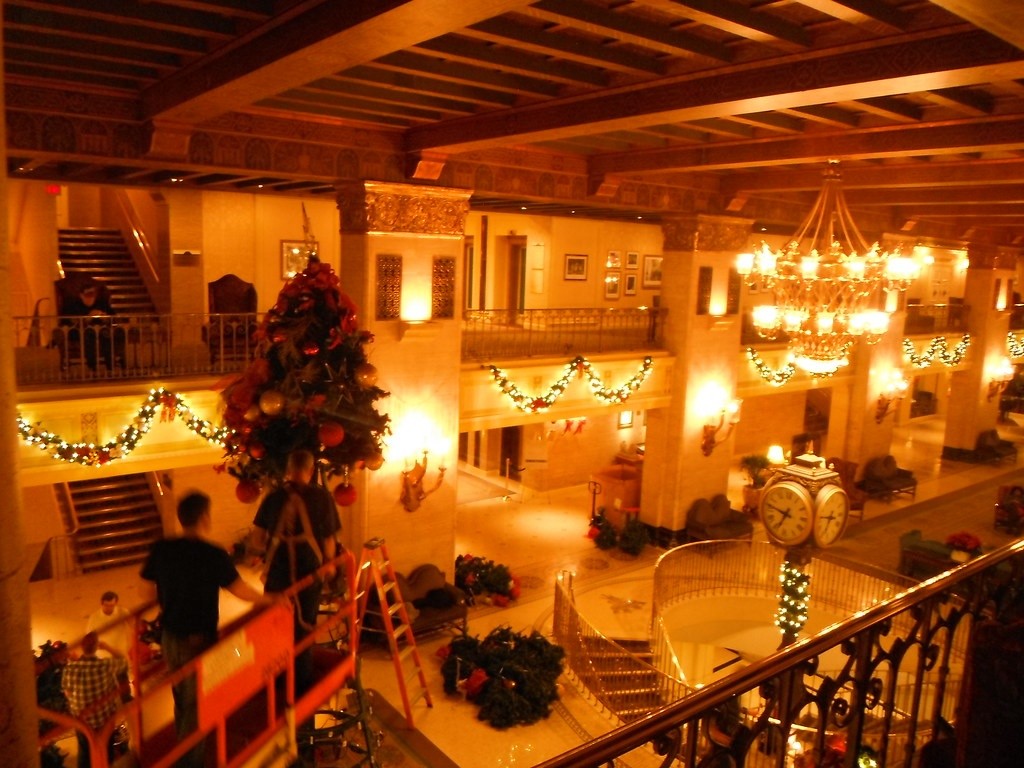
[686,494,753,558]
[865,455,916,502]
[978,430,1017,466]
[898,529,980,574]
[365,565,467,651]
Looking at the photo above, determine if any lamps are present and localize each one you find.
[732,170,906,384]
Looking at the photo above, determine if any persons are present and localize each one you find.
[60,283,125,372]
[61,631,129,768]
[86,590,137,704]
[251,449,342,698]
[140,492,274,768]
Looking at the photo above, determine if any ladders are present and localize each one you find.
[353,537,434,731]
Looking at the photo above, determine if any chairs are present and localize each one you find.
[826,458,866,518]
[947,296,965,327]
[202,274,259,372]
[907,298,934,331]
[993,484,1024,528]
[53,272,142,376]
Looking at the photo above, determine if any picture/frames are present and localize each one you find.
[642,254,663,287]
[564,254,587,280]
[625,274,637,295]
[605,271,621,299]
[605,251,622,271]
[626,251,639,270]
[279,240,320,281]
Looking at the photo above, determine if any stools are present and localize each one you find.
[300,646,378,768]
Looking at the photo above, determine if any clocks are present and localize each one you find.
[811,484,849,549]
[759,482,814,547]
